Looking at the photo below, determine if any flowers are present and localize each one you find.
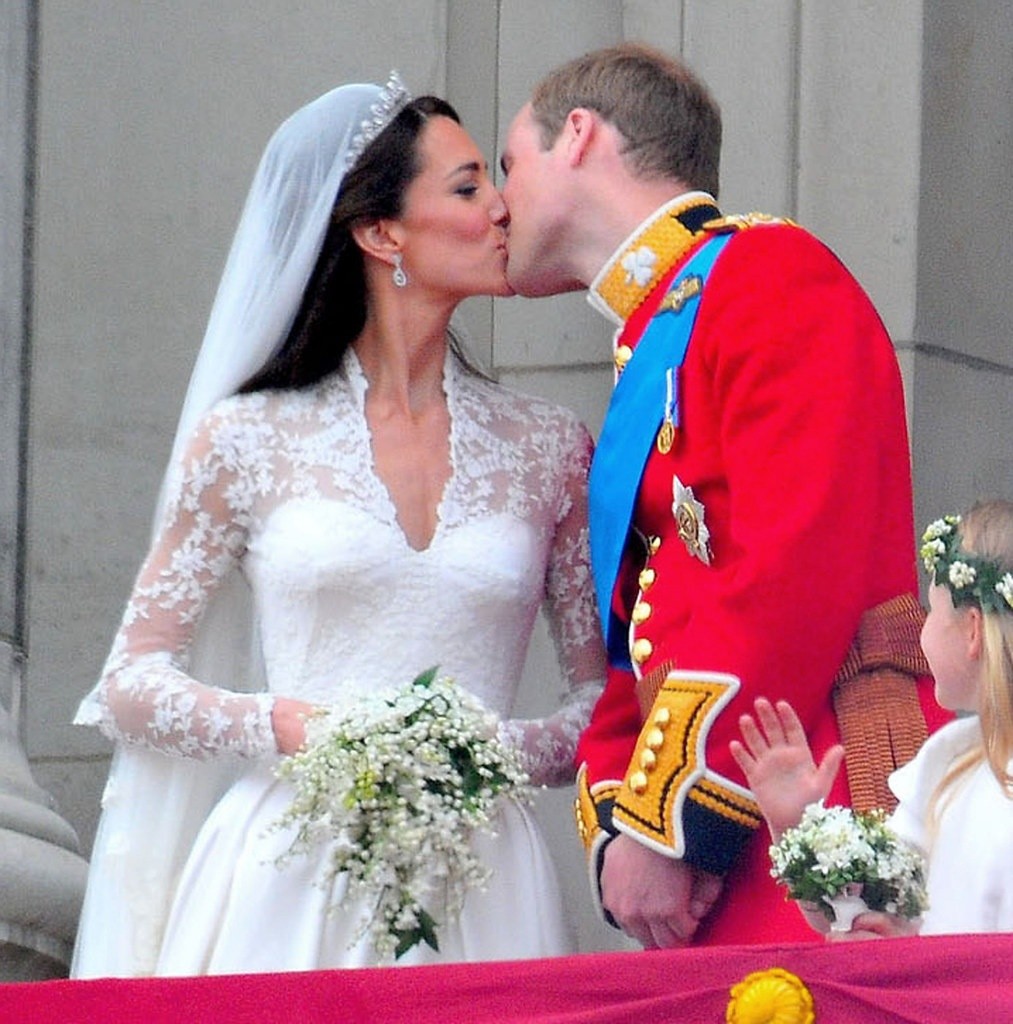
[922,516,1013,617]
[257,659,546,963]
[769,795,934,936]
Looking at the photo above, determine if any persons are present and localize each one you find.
[729,495,1013,943]
[71,73,606,981]
[502,43,955,949]
